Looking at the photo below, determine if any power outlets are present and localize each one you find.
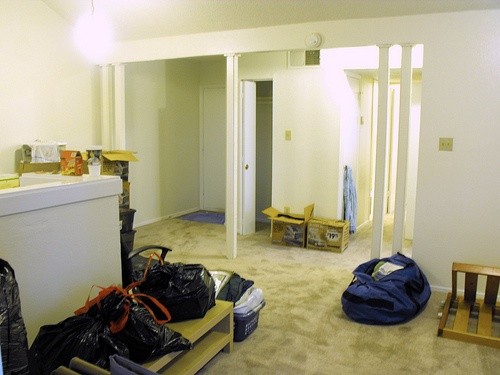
[285,130,291,140]
[439,138,453,151]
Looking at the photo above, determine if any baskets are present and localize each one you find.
[233,299,265,342]
[119,208,136,252]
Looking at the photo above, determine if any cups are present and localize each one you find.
[86,146,104,176]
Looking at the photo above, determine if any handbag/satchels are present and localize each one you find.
[341,252,431,325]
[0,258,31,375]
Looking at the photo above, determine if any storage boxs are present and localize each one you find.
[306,217,351,253]
[20,142,137,254]
[262,204,314,248]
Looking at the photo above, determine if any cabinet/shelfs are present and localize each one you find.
[142,299,234,375]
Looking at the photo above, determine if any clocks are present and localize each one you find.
[305,33,321,48]
